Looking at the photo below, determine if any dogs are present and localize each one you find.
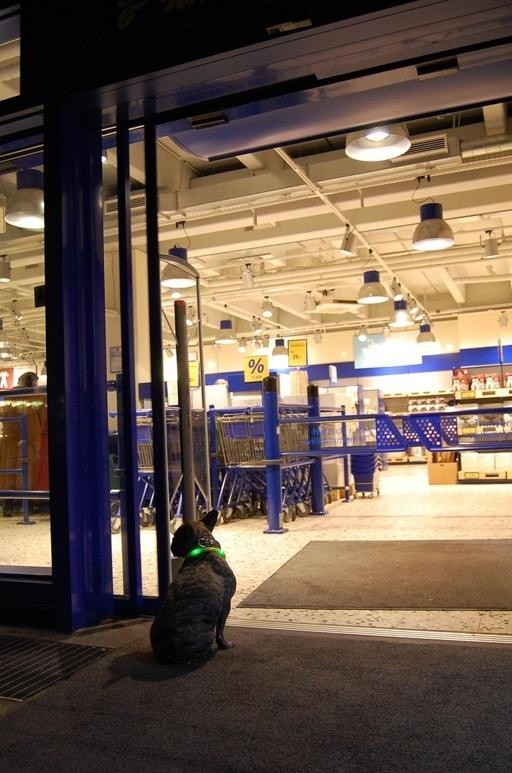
[149,509,237,670]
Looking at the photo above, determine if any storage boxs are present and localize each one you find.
[427,451,459,484]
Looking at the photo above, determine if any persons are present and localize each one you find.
[18,372,39,387]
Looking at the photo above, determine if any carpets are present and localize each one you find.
[235,540,511,611]
[0,627,512,773]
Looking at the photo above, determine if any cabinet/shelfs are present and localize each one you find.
[454,364,512,484]
[379,391,459,464]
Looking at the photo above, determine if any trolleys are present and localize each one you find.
[105,404,385,535]
